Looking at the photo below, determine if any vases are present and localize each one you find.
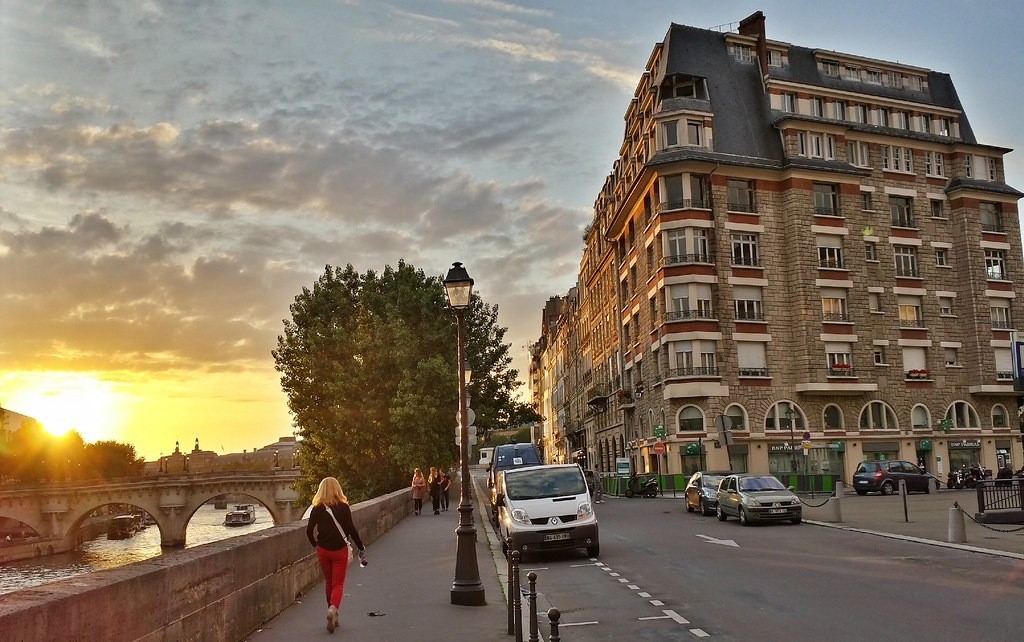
[910,373,918,377]
[842,368,849,371]
[919,373,927,377]
[833,368,841,371]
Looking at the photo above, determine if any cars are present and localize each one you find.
[684,470,740,517]
[715,472,803,528]
[496,463,600,560]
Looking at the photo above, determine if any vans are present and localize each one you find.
[487,442,546,523]
[853,460,941,496]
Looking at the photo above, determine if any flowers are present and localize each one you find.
[919,369,929,373]
[832,364,841,368]
[908,369,918,373]
[841,364,851,368]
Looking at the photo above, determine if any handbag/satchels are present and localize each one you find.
[347,541,353,565]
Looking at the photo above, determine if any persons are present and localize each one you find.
[439,468,450,512]
[412,468,426,515]
[307,477,368,633]
[594,464,604,503]
[428,466,444,515]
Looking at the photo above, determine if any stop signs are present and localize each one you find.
[652,442,665,454]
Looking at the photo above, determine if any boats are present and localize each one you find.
[107,507,156,540]
[214,495,228,510]
[224,504,256,526]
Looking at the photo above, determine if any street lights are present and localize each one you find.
[444,259,487,607]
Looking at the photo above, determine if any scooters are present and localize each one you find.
[946,464,1024,489]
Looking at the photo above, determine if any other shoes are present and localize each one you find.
[415,511,418,515]
[441,508,444,512]
[600,500,604,503]
[419,510,421,515]
[446,508,448,511]
[595,501,600,504]
[434,510,440,515]
[327,608,335,633]
[334,611,339,628]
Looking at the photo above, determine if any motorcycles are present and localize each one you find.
[625,472,659,498]
